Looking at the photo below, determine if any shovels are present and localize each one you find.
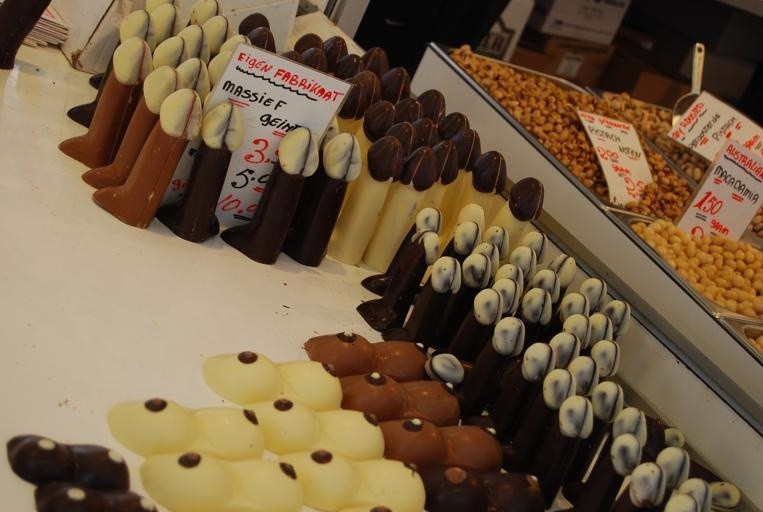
[672,43,705,125]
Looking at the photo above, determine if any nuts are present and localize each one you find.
[446,43,763,355]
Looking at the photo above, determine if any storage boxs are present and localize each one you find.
[510,0,763,110]
